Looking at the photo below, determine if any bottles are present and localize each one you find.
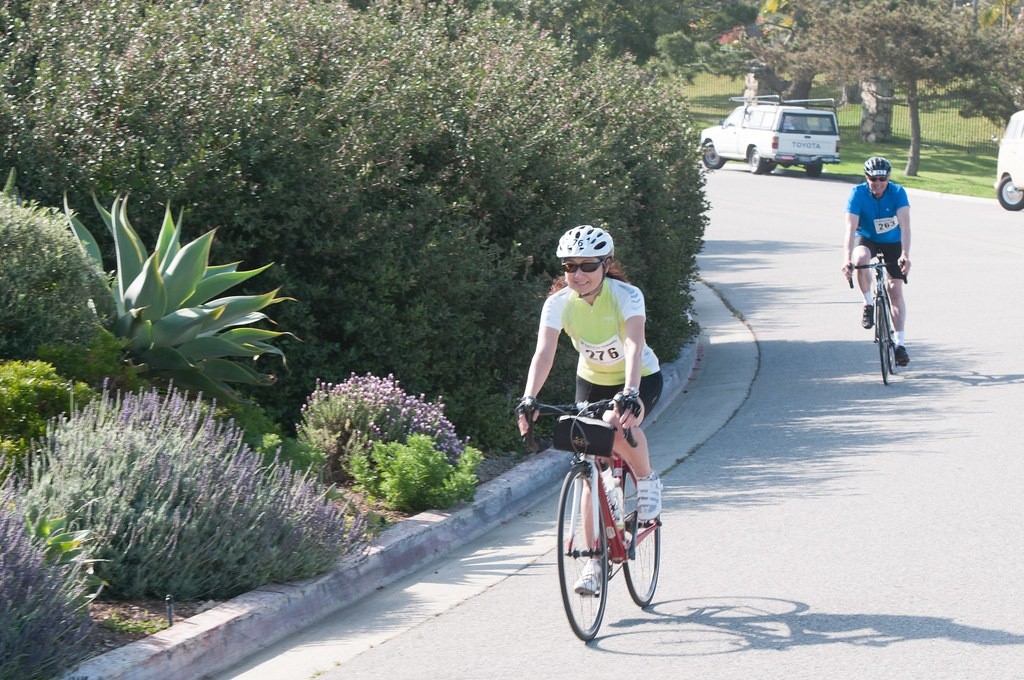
[612,476,623,527]
[599,458,619,518]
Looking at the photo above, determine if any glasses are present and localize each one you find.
[868,176,887,182]
[562,258,606,273]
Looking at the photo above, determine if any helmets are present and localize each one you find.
[864,156,891,176]
[556,224,614,258]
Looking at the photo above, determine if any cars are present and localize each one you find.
[996,110,1024,212]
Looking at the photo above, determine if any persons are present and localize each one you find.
[516,223,663,594]
[842,156,912,365]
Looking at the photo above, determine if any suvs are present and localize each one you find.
[699,95,841,177]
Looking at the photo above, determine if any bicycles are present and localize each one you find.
[848,249,908,385]
[514,395,662,641]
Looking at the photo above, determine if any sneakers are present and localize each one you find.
[636,473,663,520]
[862,303,875,329]
[574,561,601,593]
[896,345,910,366]
